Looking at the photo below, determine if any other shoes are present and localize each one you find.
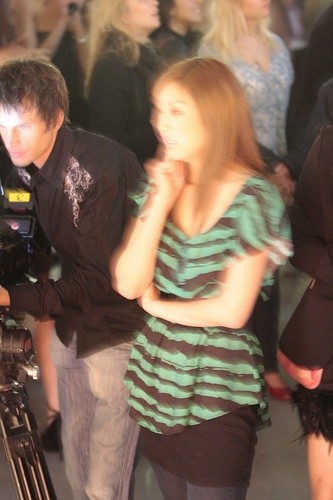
[265,373,296,402]
[41,407,64,452]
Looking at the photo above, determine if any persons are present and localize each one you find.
[112,56,296,500]
[0,59,149,500]
[197,0,296,403]
[274,124,333,500]
[0,1,66,462]
[28,1,206,124]
[255,1,332,205]
[85,0,176,165]
